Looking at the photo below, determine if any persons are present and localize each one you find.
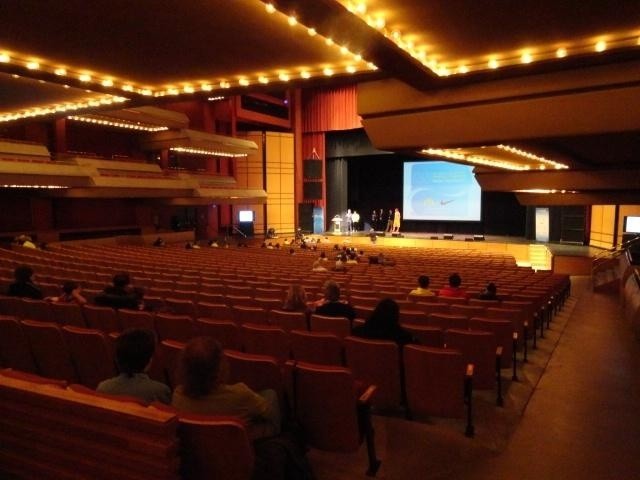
[168,336,309,480]
[351,298,422,351]
[93,272,139,311]
[185,231,248,249]
[345,207,401,233]
[478,282,500,301]
[91,331,171,406]
[313,279,360,321]
[410,275,435,296]
[439,273,470,301]
[262,227,386,271]
[155,236,164,246]
[4,264,44,301]
[282,285,313,330]
[58,280,88,304]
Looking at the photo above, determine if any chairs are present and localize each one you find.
[0,237,573,479]
